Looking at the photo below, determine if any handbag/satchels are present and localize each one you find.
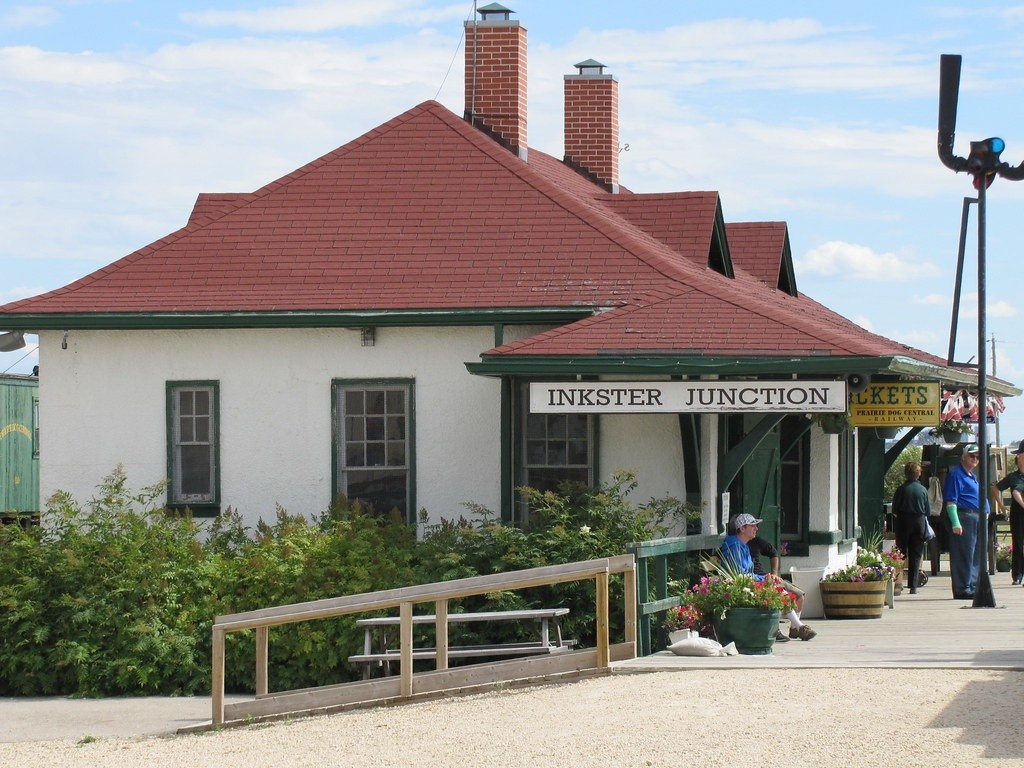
[924,516,936,543]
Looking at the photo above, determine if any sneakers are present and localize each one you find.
[789,625,817,640]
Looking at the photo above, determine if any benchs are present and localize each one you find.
[346,639,577,679]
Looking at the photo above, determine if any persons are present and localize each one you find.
[991,437,1024,588]
[709,514,792,642]
[892,462,932,594]
[722,512,817,640]
[944,444,991,600]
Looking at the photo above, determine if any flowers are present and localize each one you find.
[884,547,904,571]
[826,564,892,581]
[930,420,978,435]
[662,605,707,632]
[809,411,856,432]
[688,572,795,612]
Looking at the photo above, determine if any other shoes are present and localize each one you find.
[775,629,790,641]
[954,591,975,599]
[910,589,917,594]
[1012,579,1021,584]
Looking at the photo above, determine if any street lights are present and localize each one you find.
[968,135,1005,610]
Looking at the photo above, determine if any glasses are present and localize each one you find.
[970,454,979,460]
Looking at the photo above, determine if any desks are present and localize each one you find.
[356,608,570,678]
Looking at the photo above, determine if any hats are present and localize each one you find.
[963,445,979,454]
[1011,439,1024,454]
[735,513,762,530]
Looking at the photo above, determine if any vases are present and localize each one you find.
[713,607,780,655]
[666,628,699,644]
[893,572,903,595]
[821,418,844,434]
[820,580,887,620]
[944,432,961,443]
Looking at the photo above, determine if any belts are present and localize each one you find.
[958,508,978,513]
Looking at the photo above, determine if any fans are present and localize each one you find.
[839,371,872,394]
